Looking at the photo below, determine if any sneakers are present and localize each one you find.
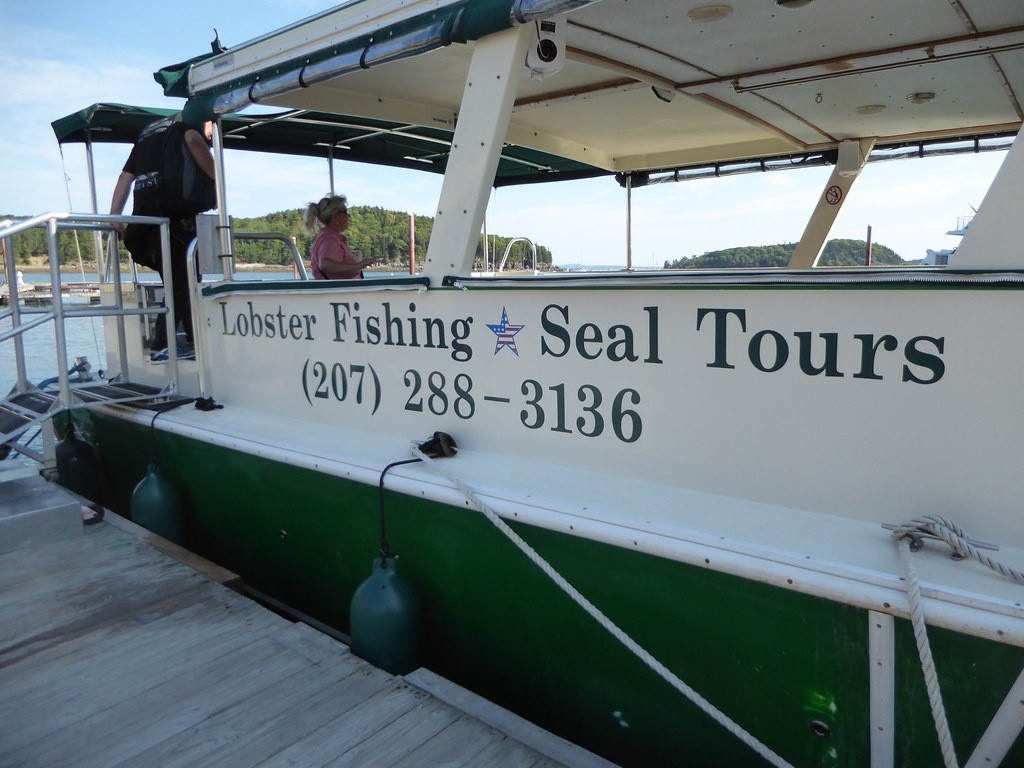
[151,346,195,364]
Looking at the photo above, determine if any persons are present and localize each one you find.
[109,113,218,365]
[304,195,379,279]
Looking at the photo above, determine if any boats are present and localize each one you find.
[1,0,1024,768]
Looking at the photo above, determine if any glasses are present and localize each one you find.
[336,209,349,214]
